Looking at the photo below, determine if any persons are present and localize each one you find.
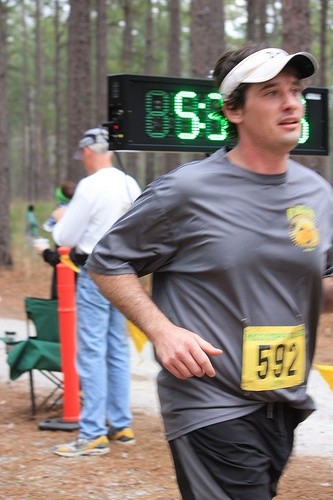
[26,182,87,299]
[51,127,142,457]
[83,44,333,500]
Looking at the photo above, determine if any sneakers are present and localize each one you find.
[107,425,136,445]
[54,436,109,456]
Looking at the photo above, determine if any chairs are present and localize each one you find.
[0,297,85,417]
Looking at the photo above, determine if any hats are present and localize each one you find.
[73,128,109,160]
[219,49,319,100]
